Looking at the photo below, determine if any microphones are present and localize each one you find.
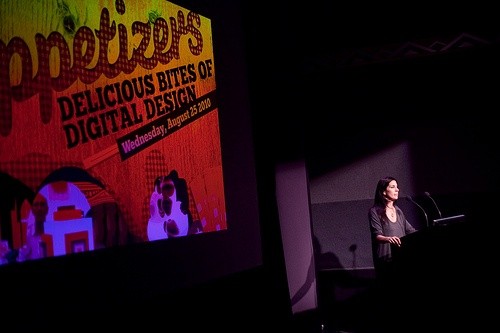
[405,194,429,228]
[425,191,442,219]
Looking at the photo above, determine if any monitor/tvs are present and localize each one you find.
[433,214,465,227]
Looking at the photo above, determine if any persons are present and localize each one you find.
[369,176,418,259]
